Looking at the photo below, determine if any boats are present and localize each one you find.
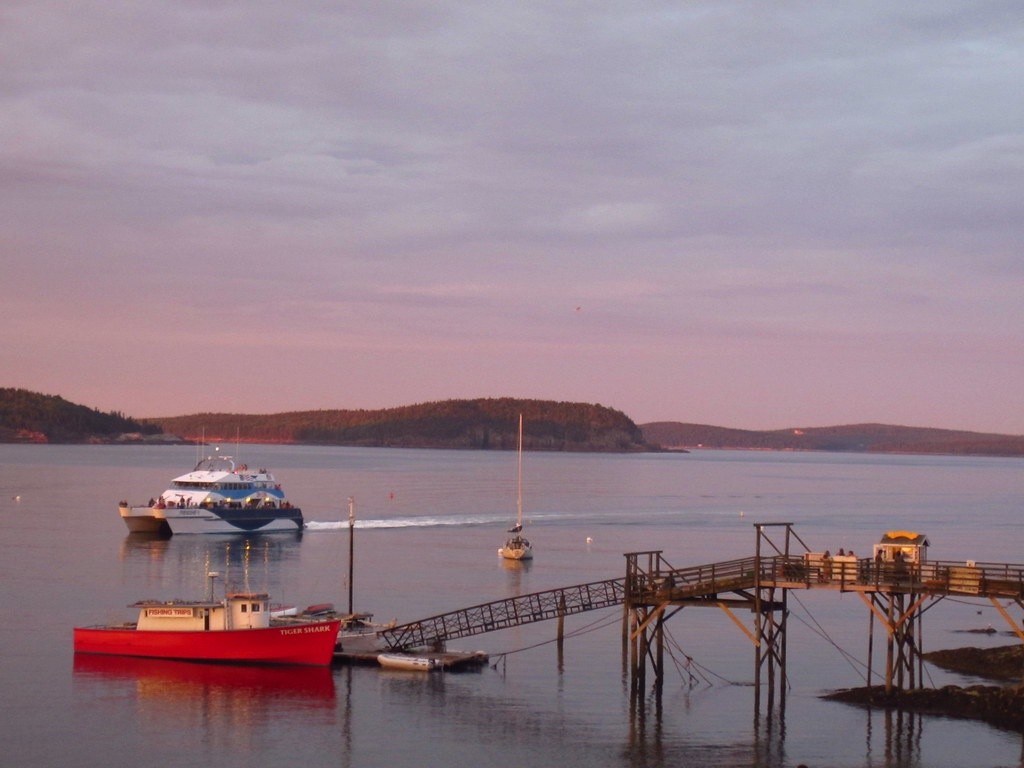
[117,422,289,534]
[72,539,343,668]
[305,601,336,616]
[376,652,490,680]
[153,497,308,535]
[271,604,298,619]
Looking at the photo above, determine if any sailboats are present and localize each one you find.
[497,413,536,561]
[331,495,430,660]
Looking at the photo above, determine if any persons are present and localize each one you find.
[201,483,221,490]
[118,500,127,508]
[259,466,268,473]
[157,495,295,509]
[837,547,845,556]
[875,550,882,585]
[275,483,281,490]
[847,551,855,557]
[240,464,247,470]
[894,551,906,586]
[148,497,156,507]
[821,550,833,580]
